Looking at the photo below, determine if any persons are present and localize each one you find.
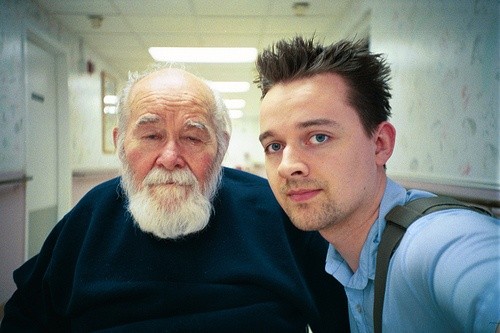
[0,65,351,333]
[253,42,499,332]
